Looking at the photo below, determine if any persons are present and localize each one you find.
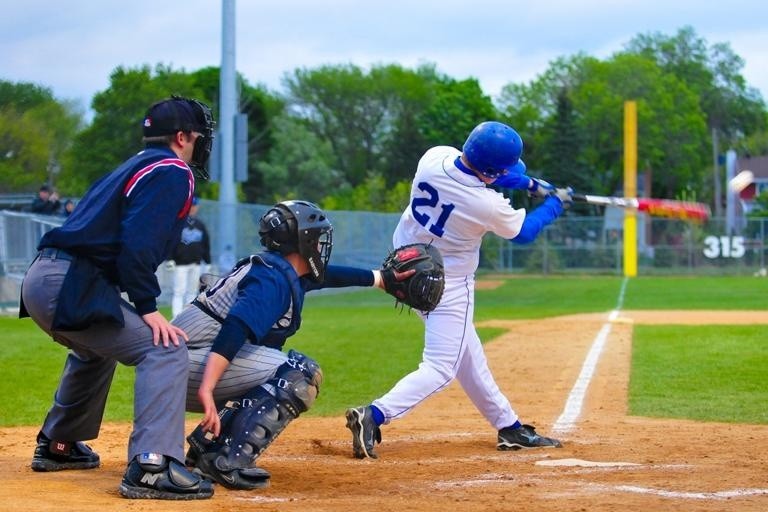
[342,118,577,461]
[164,194,213,319]
[17,95,215,501]
[63,198,74,217]
[27,184,62,218]
[166,196,419,493]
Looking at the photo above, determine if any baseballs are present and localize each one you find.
[730,169,753,192]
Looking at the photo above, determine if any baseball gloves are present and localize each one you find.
[380,244,444,311]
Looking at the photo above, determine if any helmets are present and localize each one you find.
[259,199,333,290]
[139,93,215,185]
[463,122,528,180]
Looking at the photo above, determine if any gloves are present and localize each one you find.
[527,175,578,212]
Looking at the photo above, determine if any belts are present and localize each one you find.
[39,246,76,260]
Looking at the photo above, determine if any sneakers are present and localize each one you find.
[30,437,101,470]
[185,442,271,489]
[495,423,561,450]
[119,454,214,499]
[344,403,381,463]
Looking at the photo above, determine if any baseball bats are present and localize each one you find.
[527,189,711,224]
